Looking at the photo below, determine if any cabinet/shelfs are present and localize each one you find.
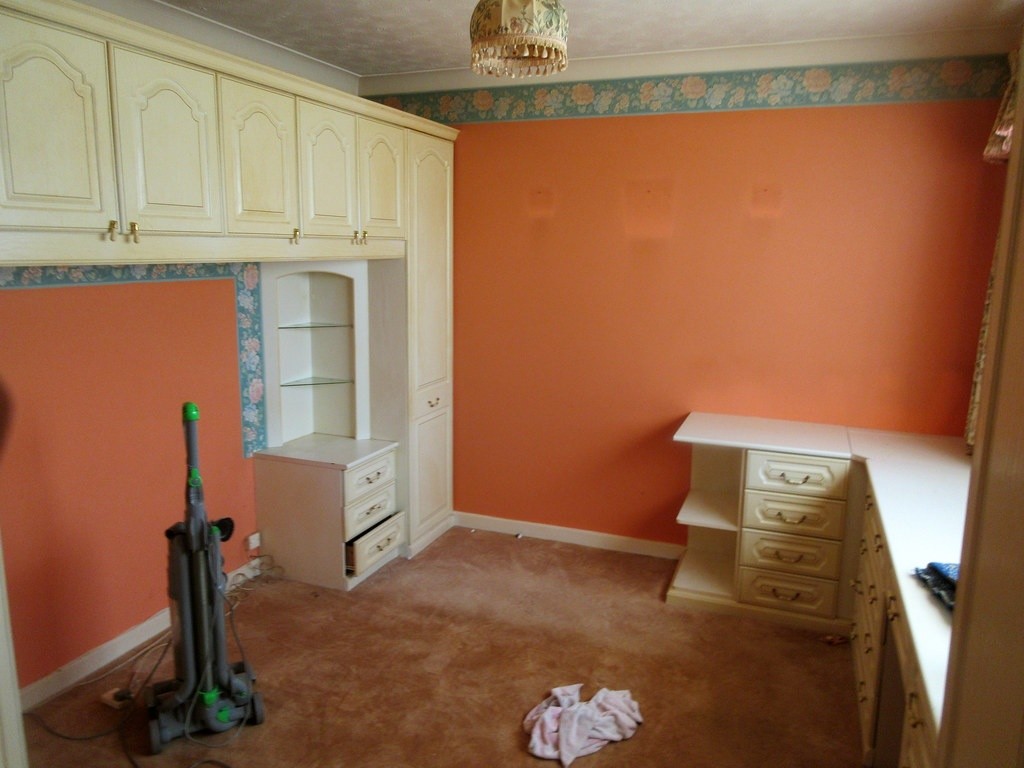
[0,7,225,236]
[261,262,370,452]
[225,85,297,241]
[342,451,407,576]
[405,137,457,557]
[731,453,851,620]
[847,494,936,767]
[297,101,404,246]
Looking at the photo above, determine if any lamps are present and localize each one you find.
[469,0,569,78]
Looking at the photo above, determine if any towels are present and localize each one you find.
[522,681,644,768]
